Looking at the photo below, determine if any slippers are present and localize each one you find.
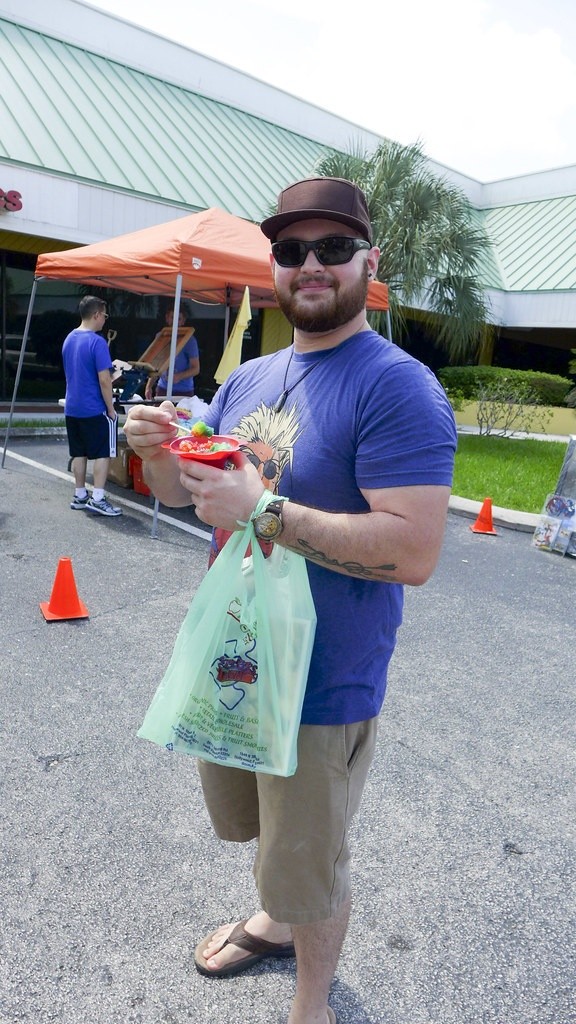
[326,1005,337,1024]
[194,915,296,976]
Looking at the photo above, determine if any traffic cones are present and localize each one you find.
[470,497,498,535]
[40,558,91,623]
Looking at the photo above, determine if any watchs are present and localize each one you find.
[250,500,285,544]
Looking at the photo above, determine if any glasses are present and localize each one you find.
[100,312,109,319]
[270,237,371,268]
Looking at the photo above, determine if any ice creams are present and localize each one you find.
[179,420,232,453]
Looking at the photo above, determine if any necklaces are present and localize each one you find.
[274,321,367,412]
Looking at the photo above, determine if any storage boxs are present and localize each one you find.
[128,455,151,497]
[108,442,133,488]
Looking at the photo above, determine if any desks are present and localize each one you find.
[57,396,204,471]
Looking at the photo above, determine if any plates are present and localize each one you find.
[161,436,242,461]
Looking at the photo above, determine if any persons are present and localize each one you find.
[124,180,461,1024]
[144,302,198,396]
[62,295,124,517]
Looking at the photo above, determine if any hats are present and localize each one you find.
[260,177,373,249]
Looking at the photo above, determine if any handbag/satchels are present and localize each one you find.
[135,489,316,779]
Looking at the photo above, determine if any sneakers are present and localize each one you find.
[70,490,92,509]
[85,496,123,516]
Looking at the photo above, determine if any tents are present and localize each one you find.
[1,205,394,476]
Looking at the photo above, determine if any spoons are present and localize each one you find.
[170,422,214,438]
